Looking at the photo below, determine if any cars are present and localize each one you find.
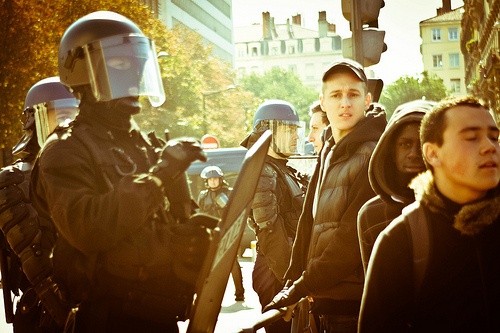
[185,146,250,201]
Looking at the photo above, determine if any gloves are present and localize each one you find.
[169,223,210,273]
[149,137,208,194]
[262,284,302,318]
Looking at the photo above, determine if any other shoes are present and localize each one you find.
[235,294,244,301]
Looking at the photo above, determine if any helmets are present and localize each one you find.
[254,100,306,156]
[23,75,81,149]
[200,166,223,178]
[58,11,166,107]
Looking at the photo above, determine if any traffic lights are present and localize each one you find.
[341,0,387,104]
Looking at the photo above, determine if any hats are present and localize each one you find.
[322,58,367,82]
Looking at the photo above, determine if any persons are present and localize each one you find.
[198,165,245,301]
[240,100,310,333]
[262,58,388,333]
[0,12,207,333]
[308,102,330,154]
[357,94,500,333]
[357,100,439,277]
